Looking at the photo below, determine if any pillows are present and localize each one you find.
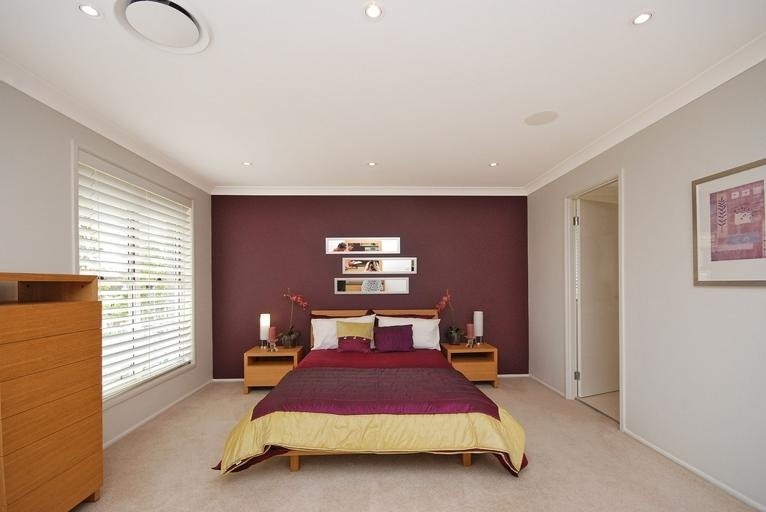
[310,313,441,353]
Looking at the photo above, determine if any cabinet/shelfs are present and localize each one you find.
[0,270,105,511]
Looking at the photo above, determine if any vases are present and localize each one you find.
[445,329,463,345]
[283,338,296,348]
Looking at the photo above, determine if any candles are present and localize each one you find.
[466,323,474,338]
[269,326,276,340]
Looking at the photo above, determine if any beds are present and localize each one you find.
[274,309,491,472]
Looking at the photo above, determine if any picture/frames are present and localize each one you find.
[325,237,418,294]
[690,157,766,288]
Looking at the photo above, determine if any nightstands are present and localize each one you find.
[441,342,498,389]
[243,345,303,394]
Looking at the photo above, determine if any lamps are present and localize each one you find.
[259,314,271,347]
[473,311,484,345]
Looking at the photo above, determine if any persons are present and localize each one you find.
[334,242,347,252]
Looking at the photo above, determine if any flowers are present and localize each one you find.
[277,287,311,345]
[433,288,461,330]
